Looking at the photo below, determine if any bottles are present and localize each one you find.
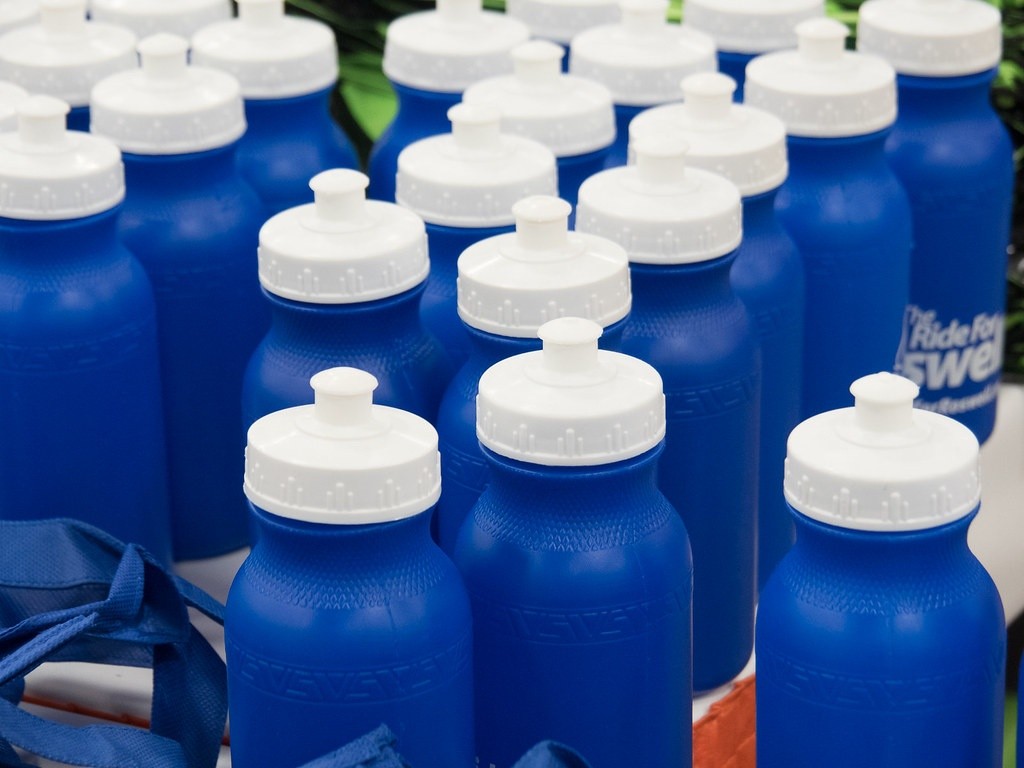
[437,194,633,534]
[452,318,693,768]
[189,0,359,217]
[368,0,529,209]
[240,168,452,552]
[0,99,173,569]
[462,41,616,232]
[573,131,761,690]
[92,0,235,71]
[745,18,910,419]
[856,0,1012,444]
[504,0,619,75]
[0,0,136,134]
[684,0,824,106]
[393,102,558,370]
[569,0,718,169]
[755,371,1006,768]
[89,34,273,559]
[228,366,473,767]
[627,73,803,605]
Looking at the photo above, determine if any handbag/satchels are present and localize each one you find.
[0,517,226,768]
